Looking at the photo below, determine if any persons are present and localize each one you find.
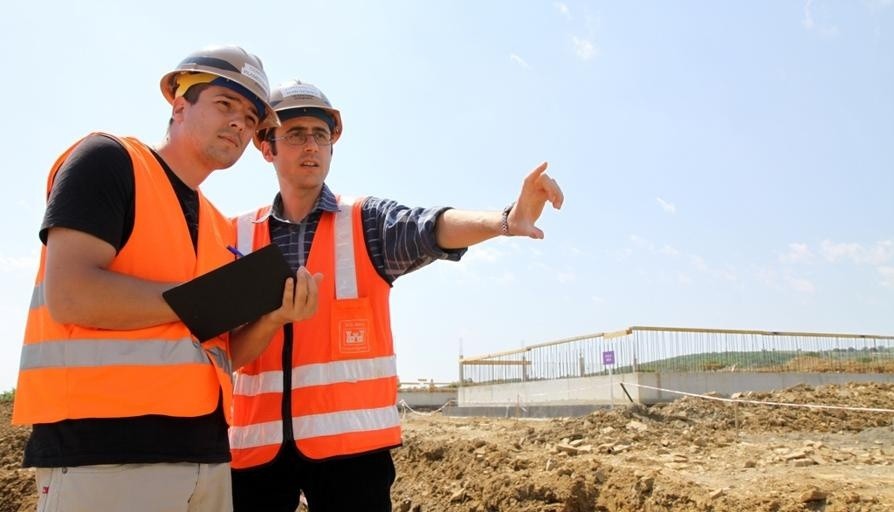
[8,43,325,511]
[225,77,565,511]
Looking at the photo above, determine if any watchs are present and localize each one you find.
[502,203,512,237]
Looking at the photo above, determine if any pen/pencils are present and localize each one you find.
[227,245,244,257]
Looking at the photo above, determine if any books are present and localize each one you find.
[160,240,297,346]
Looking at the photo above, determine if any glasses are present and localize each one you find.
[262,132,336,147]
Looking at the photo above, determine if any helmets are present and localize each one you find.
[253,82,342,146]
[161,45,281,133]
